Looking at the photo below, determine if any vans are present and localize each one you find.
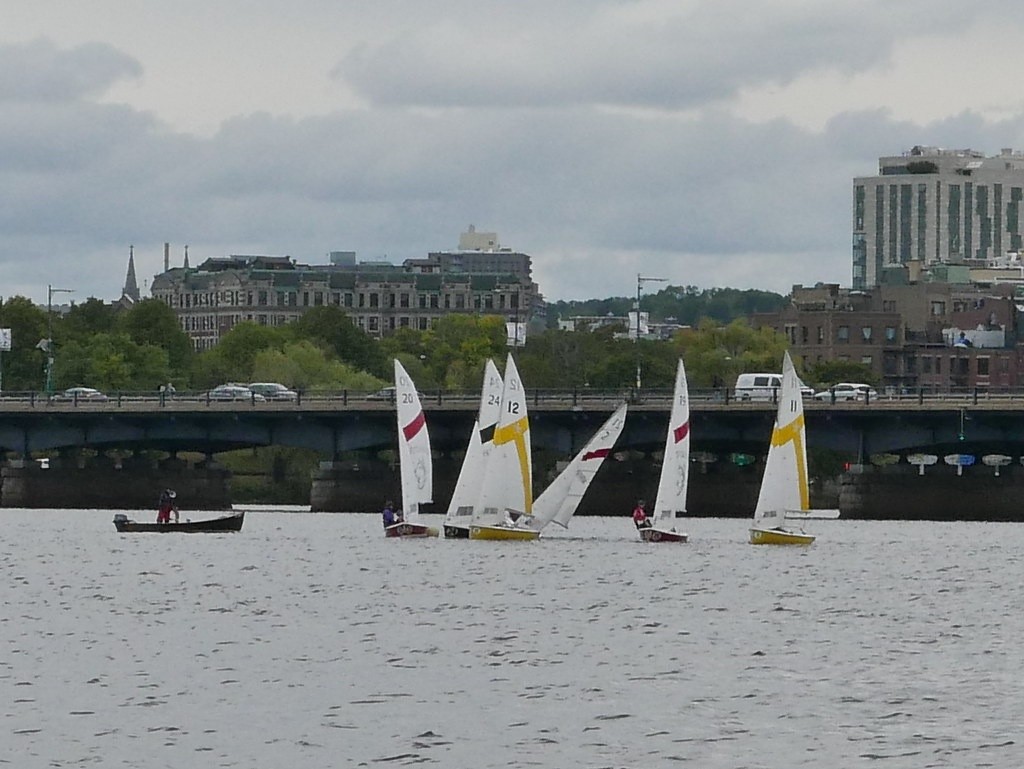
[736,373,814,402]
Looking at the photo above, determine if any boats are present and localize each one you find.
[114,511,246,533]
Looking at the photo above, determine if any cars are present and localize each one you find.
[815,382,878,403]
[51,388,108,401]
[368,386,427,400]
[199,387,267,402]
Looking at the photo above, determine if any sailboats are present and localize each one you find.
[384,358,434,538]
[750,350,816,544]
[471,351,542,540]
[517,404,628,531]
[444,360,504,538]
[640,358,690,541]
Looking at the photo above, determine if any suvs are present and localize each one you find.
[249,383,299,402]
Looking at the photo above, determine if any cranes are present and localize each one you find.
[635,273,669,309]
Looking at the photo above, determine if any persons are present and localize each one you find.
[383,501,395,528]
[158,487,172,523]
[712,376,722,402]
[158,382,166,407]
[166,488,180,523]
[633,499,651,530]
